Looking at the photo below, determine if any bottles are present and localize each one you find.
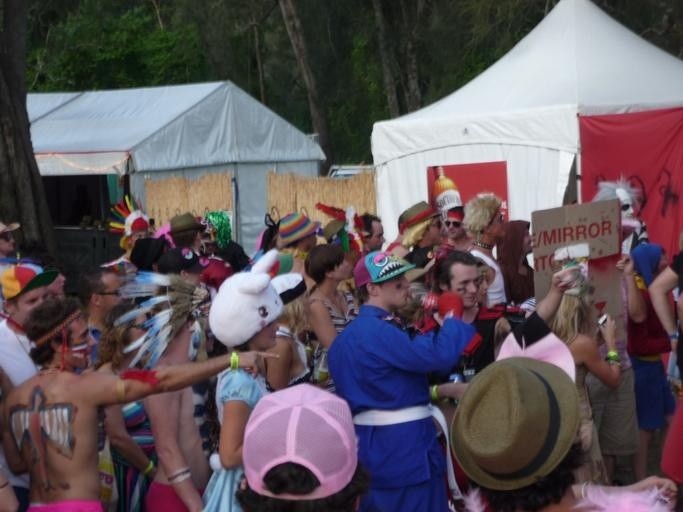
[434,165,464,229]
[304,332,314,369]
[318,349,329,383]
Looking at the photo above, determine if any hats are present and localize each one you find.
[398,201,440,229]
[210,249,307,349]
[1,263,58,300]
[447,206,464,219]
[464,193,502,232]
[353,251,416,286]
[244,385,358,502]
[449,356,579,491]
[323,219,371,244]
[475,257,497,283]
[0,223,19,231]
[278,213,321,246]
[125,211,207,274]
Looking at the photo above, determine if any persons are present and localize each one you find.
[2,182,682,511]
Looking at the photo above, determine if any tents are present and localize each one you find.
[367,0,682,268]
[22,77,328,258]
